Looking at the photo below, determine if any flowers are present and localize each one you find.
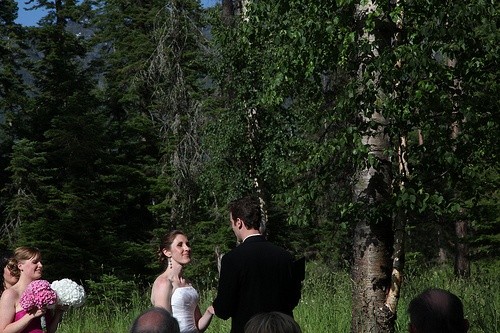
[20,278,86,333]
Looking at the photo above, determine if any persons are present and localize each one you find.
[407,289,469,333]
[242,312,303,333]
[213,195,301,333]
[130,307,180,333]
[0,247,69,333]
[151,229,214,333]
